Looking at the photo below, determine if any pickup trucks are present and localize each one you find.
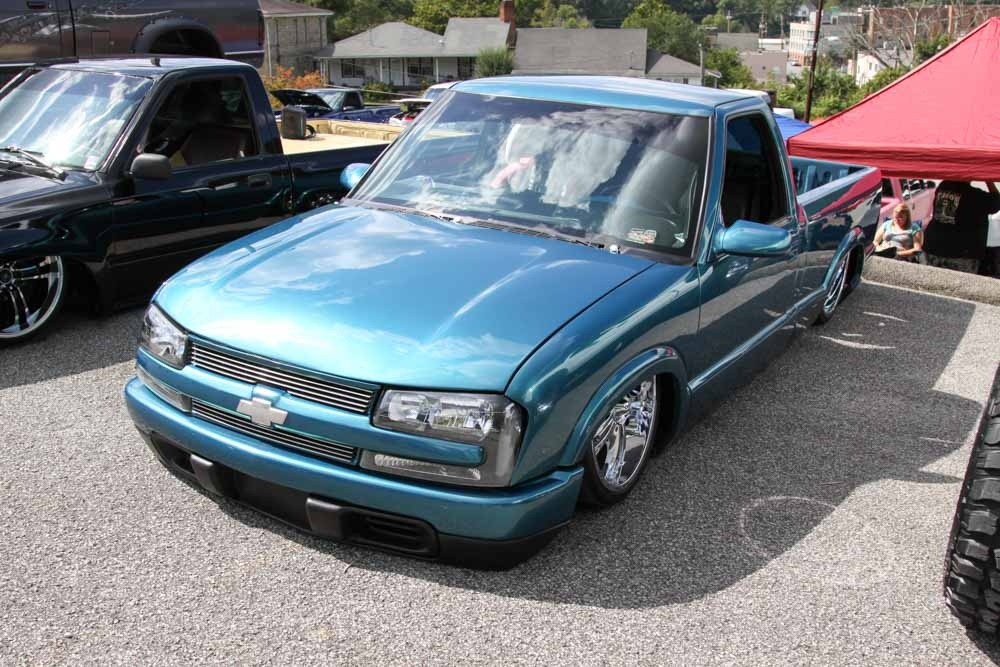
[0,53,483,350]
[0,0,267,91]
[117,75,884,573]
[267,80,466,129]
[772,112,939,258]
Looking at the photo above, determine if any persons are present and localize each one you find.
[872,205,922,257]
[408,103,421,118]
[922,180,1000,278]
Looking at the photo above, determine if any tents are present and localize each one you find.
[786,15,1000,223]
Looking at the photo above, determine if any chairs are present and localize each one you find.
[721,146,753,225]
[882,217,923,263]
[169,128,253,169]
